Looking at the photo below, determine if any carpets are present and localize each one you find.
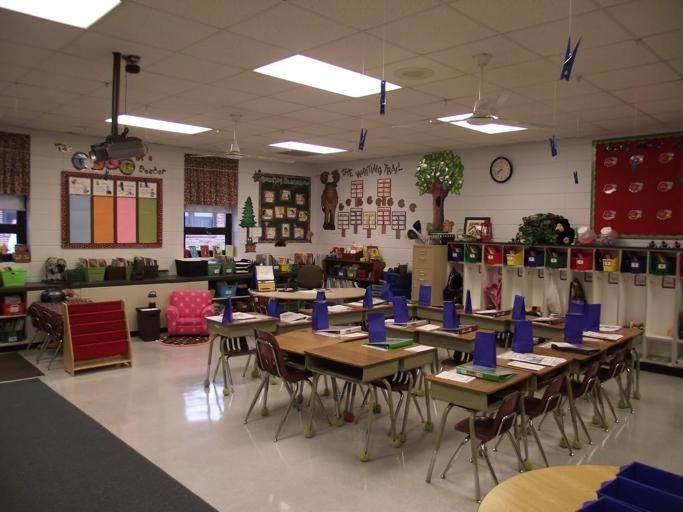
[0,377,217,512]
[160,335,211,346]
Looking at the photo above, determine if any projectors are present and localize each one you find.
[89,137,145,163]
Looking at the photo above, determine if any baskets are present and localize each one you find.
[84,267,105,282]
[0,330,24,342]
[1,303,25,315]
[1,270,27,287]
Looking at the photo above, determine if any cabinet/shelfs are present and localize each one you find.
[411,244,446,307]
[446,241,682,369]
[207,275,254,315]
[324,256,385,289]
[0,287,28,346]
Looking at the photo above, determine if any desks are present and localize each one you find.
[305,340,401,463]
[474,464,682,512]
[421,362,531,501]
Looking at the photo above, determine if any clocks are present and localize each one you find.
[490,156,513,183]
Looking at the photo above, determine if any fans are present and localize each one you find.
[191,115,294,166]
[389,53,554,134]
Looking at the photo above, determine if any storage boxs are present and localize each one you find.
[207,260,233,275]
[216,281,247,298]
[0,296,23,342]
[1,267,27,288]
[12,242,31,262]
[447,244,676,277]
[63,264,127,282]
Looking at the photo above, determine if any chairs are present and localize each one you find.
[296,264,322,290]
[494,372,576,471]
[206,285,642,441]
[441,390,529,489]
[26,300,94,373]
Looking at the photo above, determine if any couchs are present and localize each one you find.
[164,289,214,336]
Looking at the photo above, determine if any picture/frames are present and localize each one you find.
[463,217,490,241]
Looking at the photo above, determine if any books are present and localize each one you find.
[234,259,252,274]
[325,278,359,289]
[216,254,236,263]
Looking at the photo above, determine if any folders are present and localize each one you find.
[527,317,563,325]
[436,324,479,334]
[391,319,431,327]
[358,297,388,305]
[362,338,413,350]
[455,364,518,383]
[431,304,464,310]
[550,343,601,356]
[316,326,361,335]
[473,309,511,317]
